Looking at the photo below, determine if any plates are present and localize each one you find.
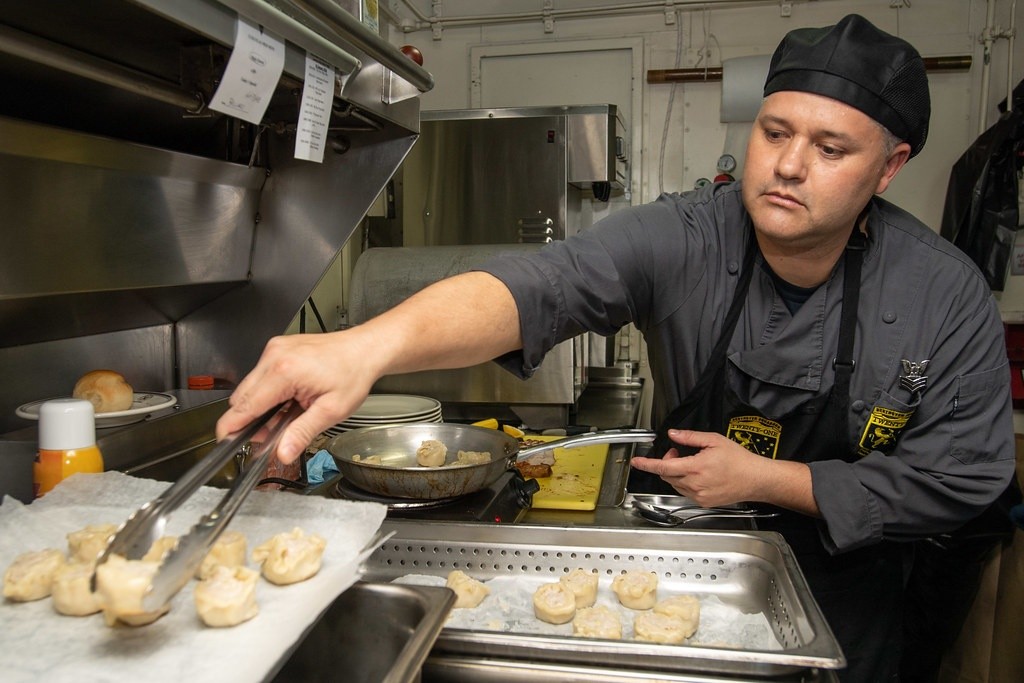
[324,393,443,439]
[15,391,177,429]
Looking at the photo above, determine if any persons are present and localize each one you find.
[216,14,1015,683]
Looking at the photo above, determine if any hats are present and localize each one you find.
[763,13,930,162]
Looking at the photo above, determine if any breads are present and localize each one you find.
[73,369,134,412]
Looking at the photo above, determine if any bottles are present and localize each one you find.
[33,399,102,495]
[188,376,214,391]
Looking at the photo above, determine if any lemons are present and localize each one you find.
[468,418,498,429]
[503,424,524,437]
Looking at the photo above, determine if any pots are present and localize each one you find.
[326,423,656,494]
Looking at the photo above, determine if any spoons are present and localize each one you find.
[631,500,756,516]
[634,510,783,525]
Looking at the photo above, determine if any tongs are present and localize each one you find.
[89,399,298,608]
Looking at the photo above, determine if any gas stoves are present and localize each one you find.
[284,463,540,524]
